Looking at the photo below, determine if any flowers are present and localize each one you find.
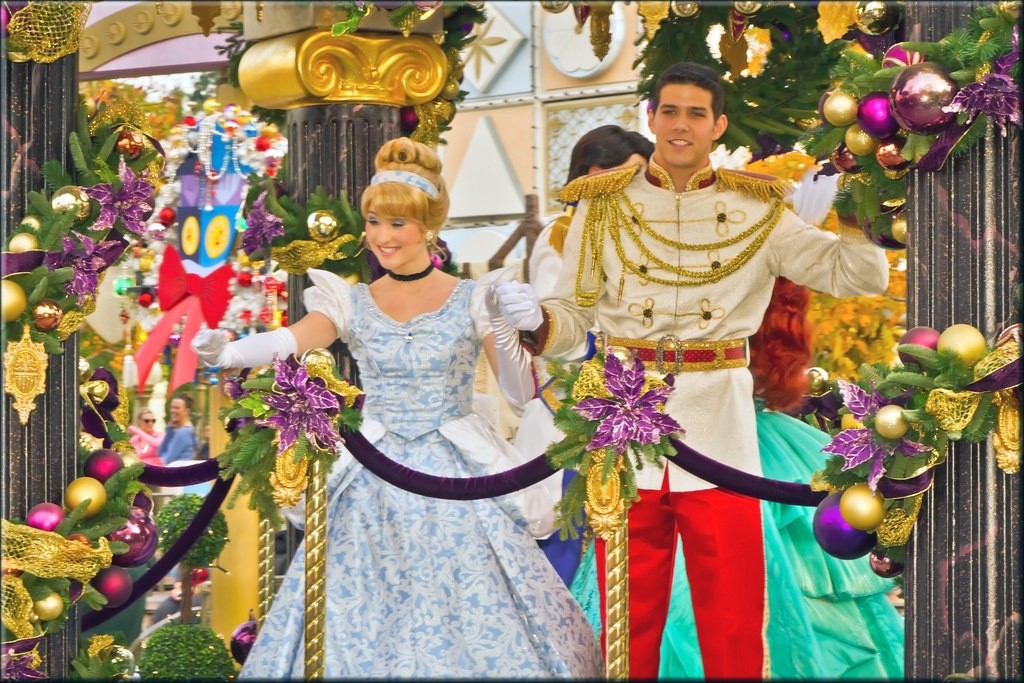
[799,332,1024,579]
[235,183,305,257]
[545,359,676,544]
[42,143,159,295]
[209,348,358,508]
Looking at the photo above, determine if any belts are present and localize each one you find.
[587,331,747,375]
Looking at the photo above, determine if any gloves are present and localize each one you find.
[794,167,843,226]
[484,277,536,408]
[188,327,297,370]
[495,279,544,332]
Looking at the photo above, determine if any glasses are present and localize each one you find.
[141,419,156,423]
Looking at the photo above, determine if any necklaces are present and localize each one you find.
[389,260,434,281]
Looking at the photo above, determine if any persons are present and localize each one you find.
[187,136,606,680]
[524,124,904,681]
[495,62,890,681]
[127,406,165,458]
[158,394,195,466]
